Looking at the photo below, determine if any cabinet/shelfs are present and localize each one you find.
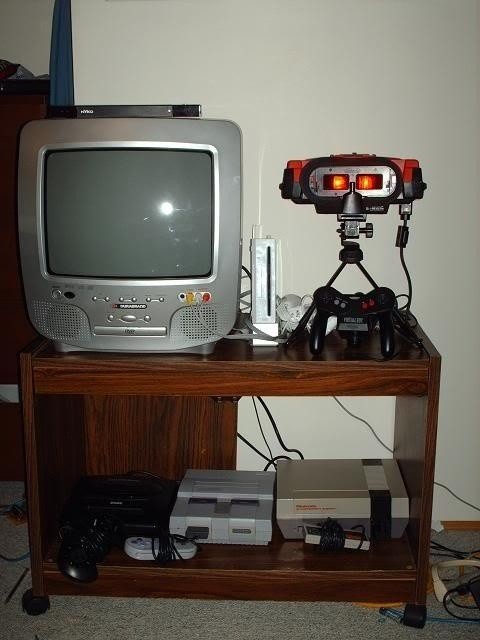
[0,79,54,482]
[19,308,441,630]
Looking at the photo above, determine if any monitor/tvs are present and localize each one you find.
[17,117,244,356]
